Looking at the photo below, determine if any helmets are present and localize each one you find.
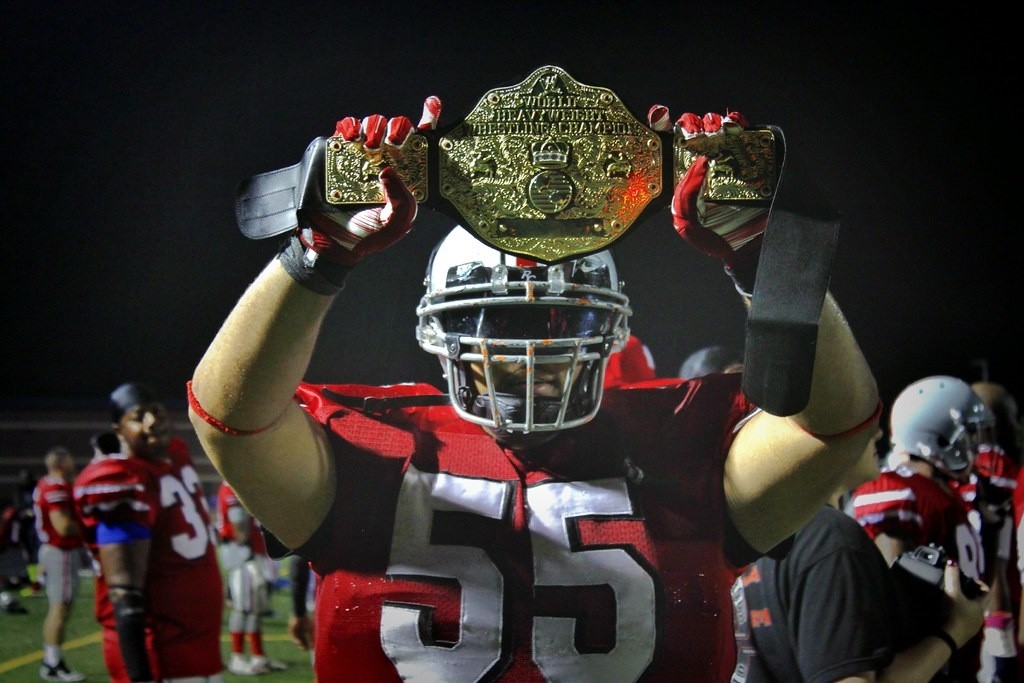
[680,345,744,381]
[888,375,996,472]
[969,381,1023,431]
[422,224,628,361]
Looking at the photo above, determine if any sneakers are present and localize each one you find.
[39,656,85,682]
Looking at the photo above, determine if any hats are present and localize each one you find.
[109,383,161,424]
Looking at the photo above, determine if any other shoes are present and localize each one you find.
[230,658,269,675]
[251,655,288,670]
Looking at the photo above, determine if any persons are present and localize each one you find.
[178,88,886,683]
[2,490,41,618]
[852,374,999,679]
[286,547,319,656]
[70,382,228,683]
[209,463,282,676]
[732,376,1000,682]
[26,446,89,681]
[954,374,1024,681]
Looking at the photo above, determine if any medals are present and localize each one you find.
[235,65,844,418]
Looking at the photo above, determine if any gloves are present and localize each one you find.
[278,95,441,297]
[647,103,775,298]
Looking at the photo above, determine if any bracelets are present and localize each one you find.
[180,378,282,440]
[780,389,883,438]
